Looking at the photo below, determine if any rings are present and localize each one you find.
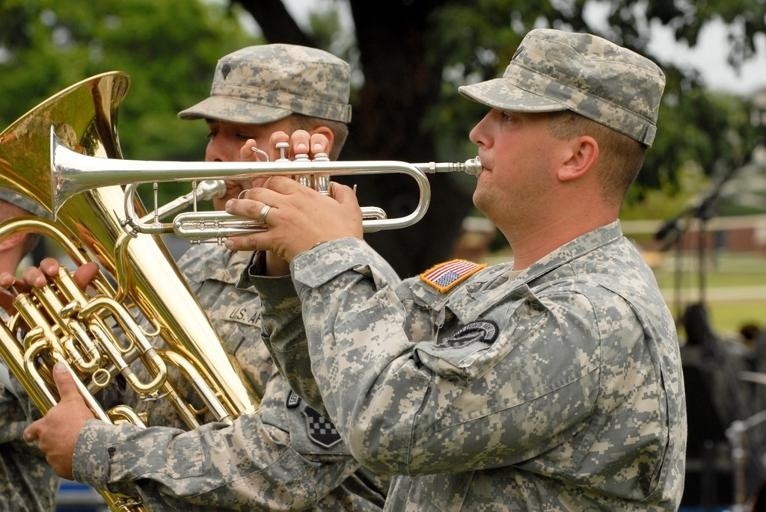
[257,204,270,223]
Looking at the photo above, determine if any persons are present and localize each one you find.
[673,298,745,467]
[0,196,134,510]
[0,39,400,509]
[736,321,766,376]
[222,25,693,510]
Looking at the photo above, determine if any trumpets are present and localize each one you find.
[50,124,482,246]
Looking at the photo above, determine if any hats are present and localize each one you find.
[176,43,353,128]
[456,28,667,148]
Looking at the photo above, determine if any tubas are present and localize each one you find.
[0,70,260,508]
